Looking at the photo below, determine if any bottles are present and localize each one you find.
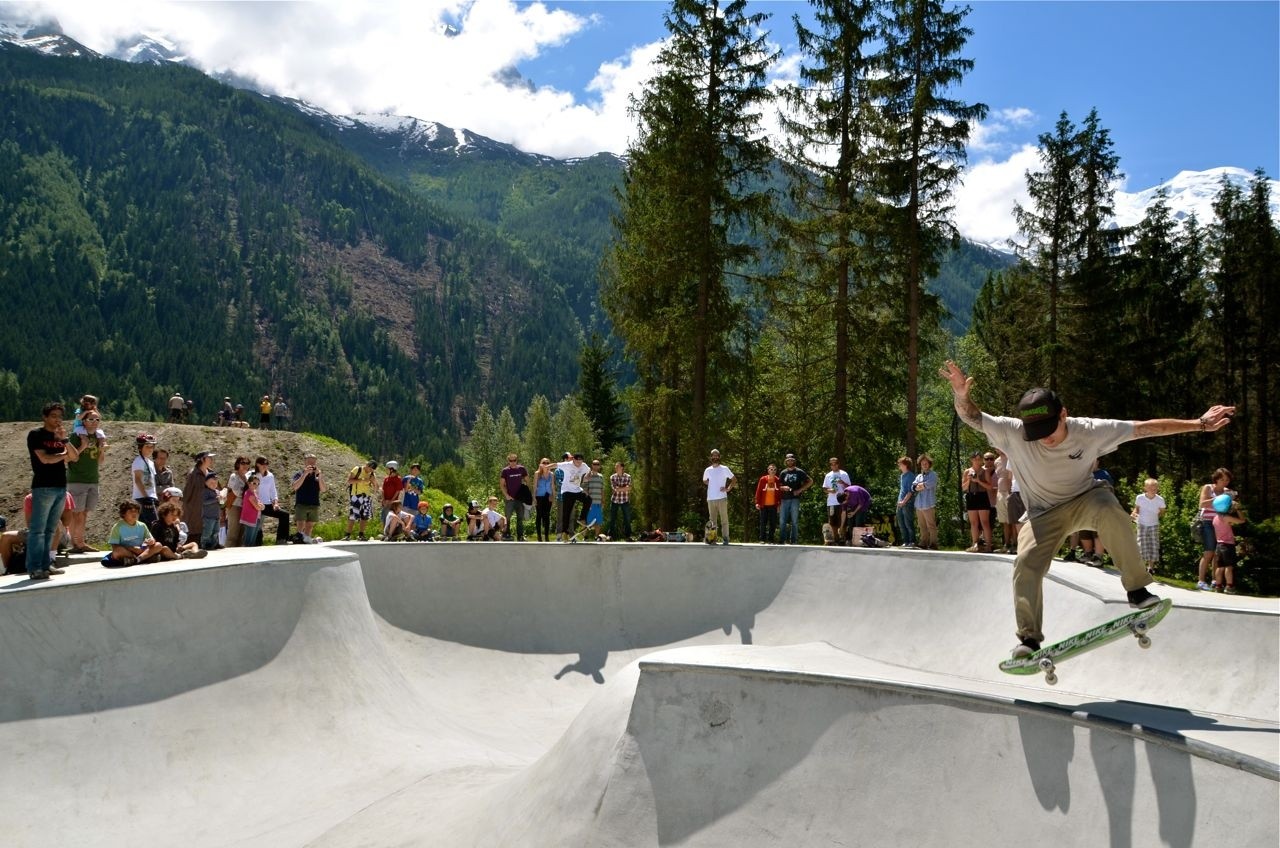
[220,488,227,504]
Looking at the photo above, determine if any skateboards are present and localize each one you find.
[567,518,598,544]
[998,597,1174,684]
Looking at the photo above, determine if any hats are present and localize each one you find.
[368,459,379,469]
[562,452,574,458]
[196,449,216,459]
[136,433,158,445]
[784,453,796,460]
[204,471,215,481]
[1016,386,1064,441]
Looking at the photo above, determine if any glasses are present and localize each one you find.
[239,460,272,486]
[983,457,996,463]
[506,458,601,470]
[86,417,101,422]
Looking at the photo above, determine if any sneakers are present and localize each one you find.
[114,529,324,566]
[706,531,851,548]
[1198,578,1240,594]
[348,528,638,543]
[1127,587,1161,610]
[895,537,1158,576]
[1011,638,1042,659]
[25,542,100,580]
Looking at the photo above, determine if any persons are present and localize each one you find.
[341,460,381,541]
[911,454,938,550]
[755,463,781,544]
[822,457,852,546]
[131,435,161,538]
[184,451,291,549]
[895,456,919,547]
[108,500,162,566]
[383,452,632,542]
[162,487,207,559]
[292,454,326,543]
[703,449,738,546]
[837,484,871,547]
[168,391,288,430]
[938,359,1247,655]
[151,449,174,495]
[778,454,814,545]
[153,503,180,561]
[25,395,106,581]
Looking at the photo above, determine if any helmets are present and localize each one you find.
[387,460,400,469]
[160,488,185,501]
[417,497,486,514]
